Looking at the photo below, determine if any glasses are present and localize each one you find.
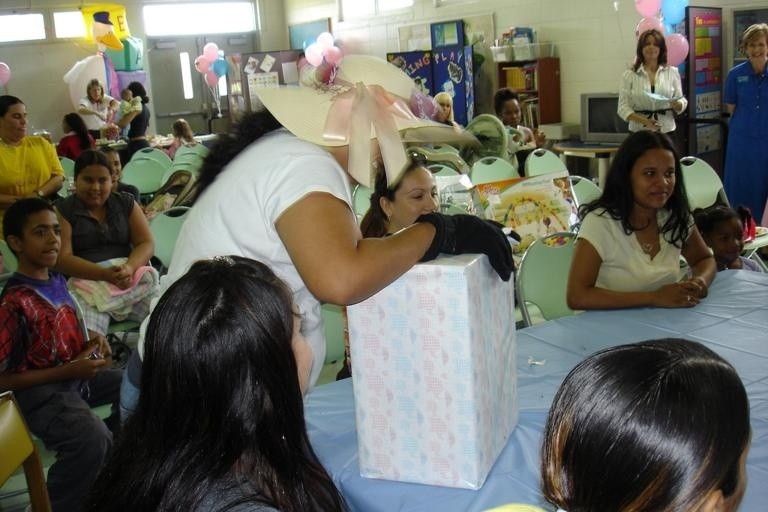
[406,151,427,162]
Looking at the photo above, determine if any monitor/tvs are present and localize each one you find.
[579,92,634,145]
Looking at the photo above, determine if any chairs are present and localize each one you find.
[679,155,767,270]
[0,142,215,422]
[313,303,351,387]
[353,115,603,328]
[1,392,56,512]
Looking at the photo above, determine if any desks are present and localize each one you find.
[304,261,766,512]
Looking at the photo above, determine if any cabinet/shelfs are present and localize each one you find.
[498,56,563,132]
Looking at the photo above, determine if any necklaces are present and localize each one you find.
[634,227,659,252]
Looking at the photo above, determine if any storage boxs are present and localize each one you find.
[488,45,513,62]
[344,242,518,493]
[512,41,555,62]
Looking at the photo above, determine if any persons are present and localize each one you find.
[616,28,689,133]
[692,203,764,272]
[564,130,718,309]
[482,335,754,512]
[723,21,768,226]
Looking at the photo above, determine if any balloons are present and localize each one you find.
[194,32,342,87]
[633,0,689,66]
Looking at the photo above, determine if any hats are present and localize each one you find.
[259,54,479,189]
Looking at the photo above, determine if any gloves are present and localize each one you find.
[414,212,521,282]
